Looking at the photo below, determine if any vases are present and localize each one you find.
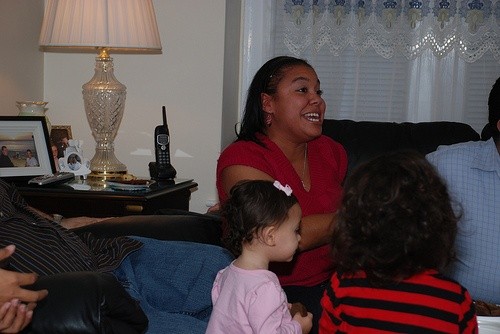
[17,101,51,134]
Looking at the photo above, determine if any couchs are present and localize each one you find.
[19,215,224,334]
[322,120,490,169]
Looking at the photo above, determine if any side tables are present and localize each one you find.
[16,175,198,218]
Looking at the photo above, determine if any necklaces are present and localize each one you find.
[301,144,308,187]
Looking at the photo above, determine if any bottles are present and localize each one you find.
[15,100,51,137]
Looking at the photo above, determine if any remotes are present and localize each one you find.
[28,172,75,186]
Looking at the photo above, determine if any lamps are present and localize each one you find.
[40,0,163,178]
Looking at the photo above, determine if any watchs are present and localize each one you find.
[51,213,64,224]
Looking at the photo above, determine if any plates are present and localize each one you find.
[105,180,155,191]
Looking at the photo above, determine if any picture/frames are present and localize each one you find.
[50,126,73,173]
[0,116,57,181]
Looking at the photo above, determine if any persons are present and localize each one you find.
[319,149,481,334]
[0,180,317,334]
[425,77,500,305]
[0,136,81,171]
[216,57,352,334]
